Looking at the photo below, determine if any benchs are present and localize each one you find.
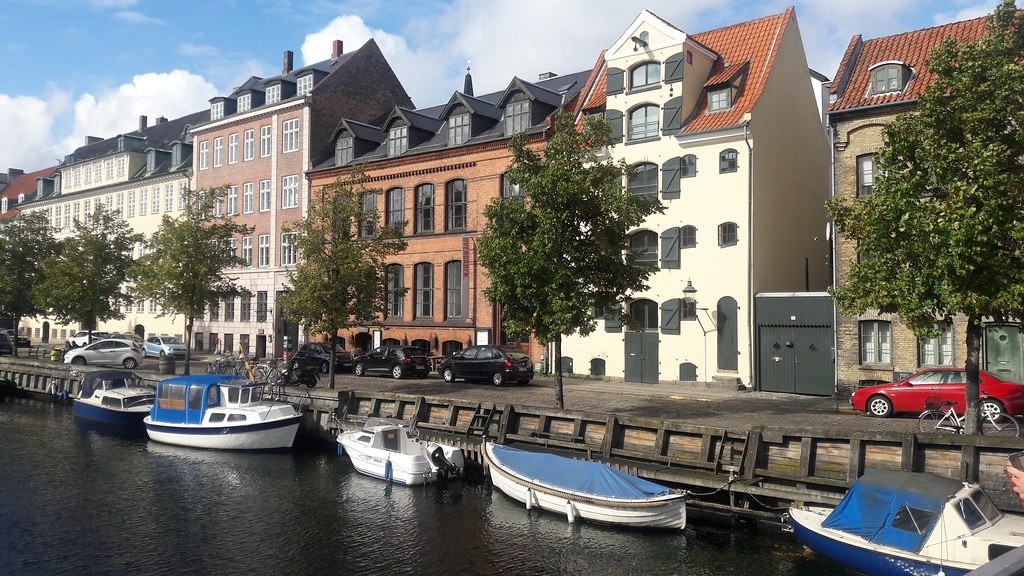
[27,345,42,358]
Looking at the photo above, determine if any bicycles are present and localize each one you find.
[917,385,1022,439]
[202,348,321,388]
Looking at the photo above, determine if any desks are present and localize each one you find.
[429,355,445,372]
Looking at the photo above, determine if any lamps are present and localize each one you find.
[683,278,698,308]
[269,335,273,342]
[631,35,646,51]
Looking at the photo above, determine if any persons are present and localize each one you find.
[1005,466,1024,508]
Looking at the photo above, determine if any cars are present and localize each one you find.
[437,344,535,387]
[352,344,431,380]
[0,331,16,356]
[847,366,1024,424]
[142,335,187,359]
[105,332,143,346]
[62,339,143,370]
[3,329,31,348]
[288,341,353,372]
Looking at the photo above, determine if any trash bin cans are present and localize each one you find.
[50,348,61,361]
[159,356,175,374]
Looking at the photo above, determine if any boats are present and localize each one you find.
[787,470,1024,576]
[72,366,154,428]
[142,375,305,448]
[0,376,17,398]
[480,438,688,531]
[335,417,466,484]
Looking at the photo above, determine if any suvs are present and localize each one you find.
[66,330,108,348]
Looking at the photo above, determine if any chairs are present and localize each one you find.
[155,339,160,344]
